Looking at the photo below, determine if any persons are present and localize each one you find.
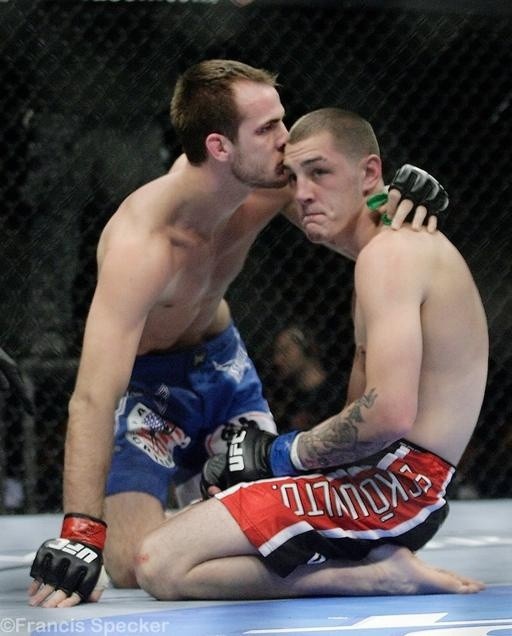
[133,107,489,602]
[268,324,346,436]
[27,60,451,608]
